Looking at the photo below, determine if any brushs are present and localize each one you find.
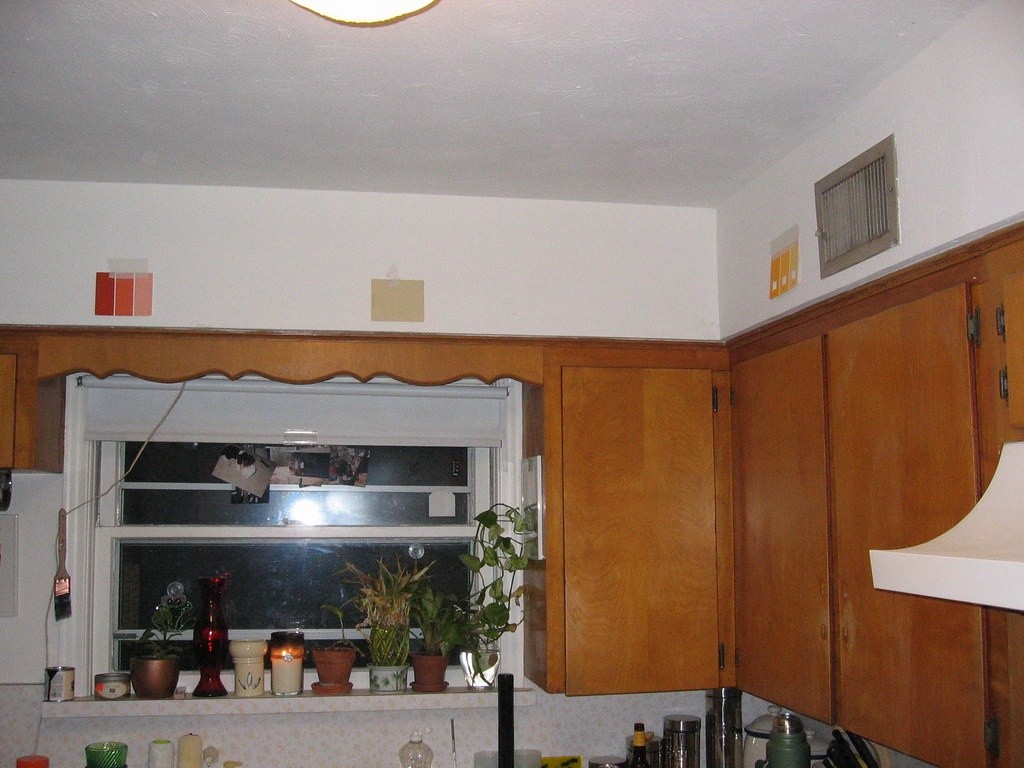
[52,507,72,621]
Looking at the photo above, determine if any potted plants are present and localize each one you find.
[407,590,459,694]
[441,500,541,692]
[309,604,365,693]
[130,582,198,697]
[345,551,437,694]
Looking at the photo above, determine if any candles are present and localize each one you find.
[269,632,306,694]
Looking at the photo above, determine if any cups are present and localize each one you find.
[704,686,745,768]
[661,714,701,768]
[268,630,306,695]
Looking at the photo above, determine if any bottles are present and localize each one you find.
[765,712,812,768]
[625,722,651,768]
[398,726,435,768]
[744,705,782,768]
[192,577,229,696]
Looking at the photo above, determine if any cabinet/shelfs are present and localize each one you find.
[543,224,1023,768]
